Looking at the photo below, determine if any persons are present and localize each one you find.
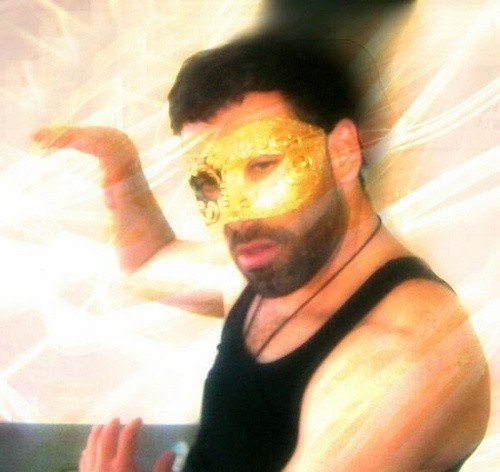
[29,31,490,471]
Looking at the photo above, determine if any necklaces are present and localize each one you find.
[240,213,382,358]
